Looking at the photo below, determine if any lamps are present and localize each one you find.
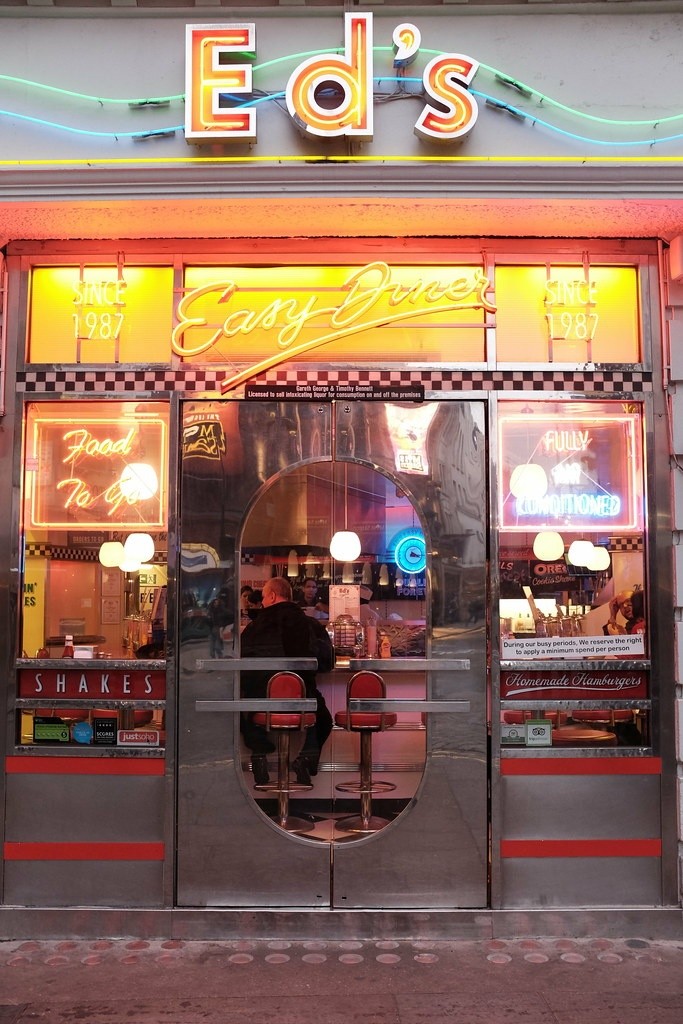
[510,464,611,571]
[99,462,159,573]
[288,550,416,587]
[329,458,361,563]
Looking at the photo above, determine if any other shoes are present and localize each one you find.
[292,756,311,784]
[250,751,270,784]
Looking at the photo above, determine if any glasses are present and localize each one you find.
[617,598,633,611]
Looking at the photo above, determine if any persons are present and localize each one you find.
[240,576,403,619]
[241,577,338,785]
[602,589,646,635]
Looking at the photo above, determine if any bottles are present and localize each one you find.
[98,652,112,659]
[516,613,532,630]
[62,635,74,659]
[381,636,391,658]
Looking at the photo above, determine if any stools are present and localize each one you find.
[254,671,316,833]
[504,707,648,748]
[335,671,397,834]
[35,707,153,728]
